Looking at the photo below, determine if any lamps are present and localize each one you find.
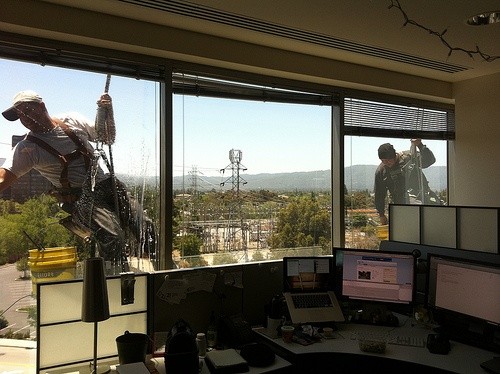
[82,257,110,374]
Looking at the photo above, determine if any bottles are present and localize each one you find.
[207,310,218,348]
[195,332,206,355]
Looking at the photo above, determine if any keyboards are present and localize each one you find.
[434,327,500,353]
[350,330,424,348]
[343,308,399,327]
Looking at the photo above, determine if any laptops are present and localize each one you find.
[282,257,346,323]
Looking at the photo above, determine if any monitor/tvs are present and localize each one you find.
[333,247,413,308]
[427,252,500,335]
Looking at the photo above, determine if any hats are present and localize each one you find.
[2,91,43,121]
[378,143,396,159]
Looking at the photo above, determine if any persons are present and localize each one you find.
[0,90,159,260]
[374,139,444,225]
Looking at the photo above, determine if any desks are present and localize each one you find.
[145,349,292,374]
[251,323,500,374]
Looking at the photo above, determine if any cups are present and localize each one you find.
[280,326,295,343]
